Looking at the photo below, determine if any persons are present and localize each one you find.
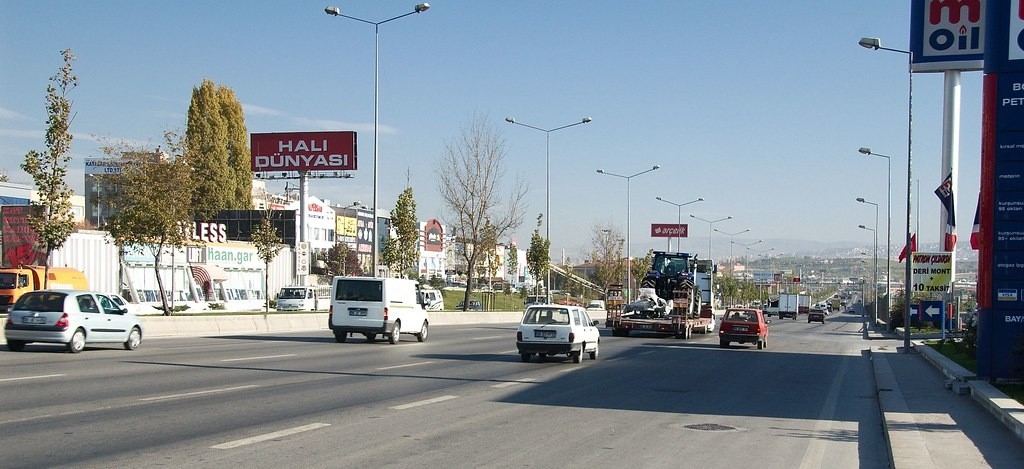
[735,312,739,317]
[546,310,557,322]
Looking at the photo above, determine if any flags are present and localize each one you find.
[898,233,916,263]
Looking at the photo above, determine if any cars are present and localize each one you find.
[455,300,482,310]
[515,304,602,364]
[3,288,146,352]
[719,307,771,350]
[524,294,605,310]
[724,291,855,324]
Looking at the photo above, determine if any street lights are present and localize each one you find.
[730,240,784,309]
[690,214,733,260]
[859,37,914,355]
[656,197,706,253]
[324,2,431,280]
[596,164,661,307]
[860,144,892,333]
[713,229,751,308]
[505,116,594,305]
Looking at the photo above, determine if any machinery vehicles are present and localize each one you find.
[0,267,91,312]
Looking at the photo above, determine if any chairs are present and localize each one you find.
[295,292,300,296]
[539,316,549,323]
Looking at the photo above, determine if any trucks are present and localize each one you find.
[275,285,331,312]
[611,250,720,340]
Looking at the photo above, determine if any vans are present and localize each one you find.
[328,276,429,345]
[415,289,445,312]
